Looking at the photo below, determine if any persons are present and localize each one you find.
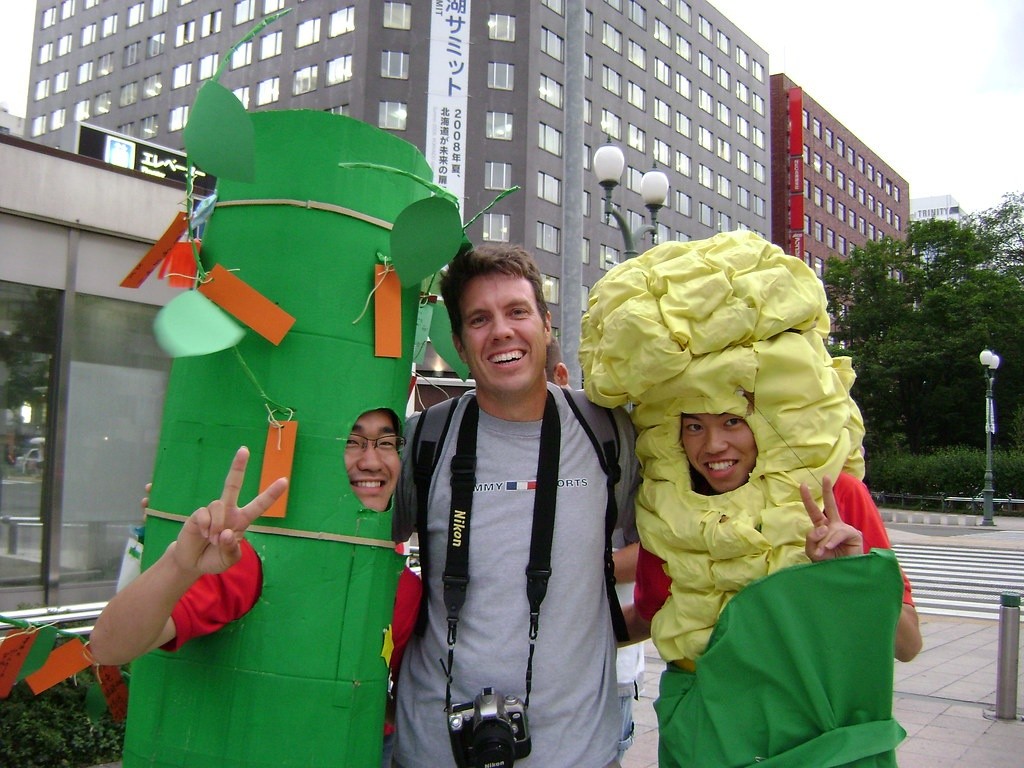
[91,7,923,768]
[142,243,865,768]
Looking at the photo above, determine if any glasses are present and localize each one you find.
[346,434,407,457]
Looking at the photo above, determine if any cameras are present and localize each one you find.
[447,686,532,768]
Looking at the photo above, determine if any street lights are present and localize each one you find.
[980,348,1001,528]
